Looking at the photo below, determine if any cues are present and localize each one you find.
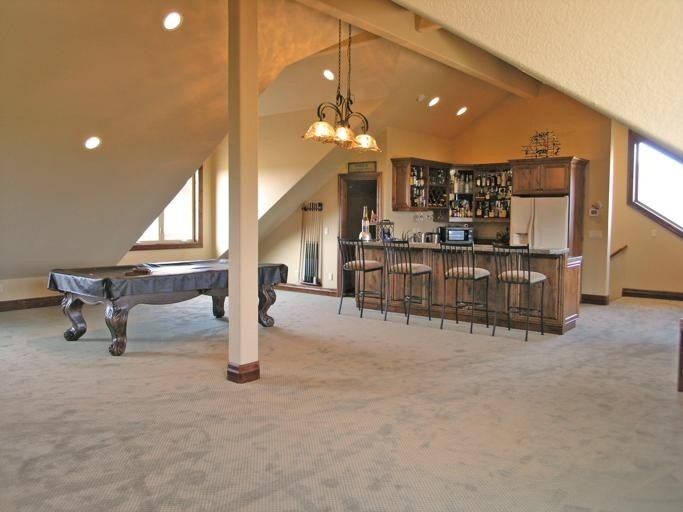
[302,202,322,283]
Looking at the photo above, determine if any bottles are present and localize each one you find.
[475,172,512,200]
[360,205,369,241]
[476,201,510,218]
[448,199,473,218]
[450,173,473,194]
[409,167,447,206]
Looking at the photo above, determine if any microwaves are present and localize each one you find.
[439,226,476,246]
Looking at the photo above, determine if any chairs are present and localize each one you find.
[489,238,548,342]
[332,234,383,320]
[437,236,488,332]
[381,237,434,323]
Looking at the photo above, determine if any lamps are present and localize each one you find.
[300,17,381,153]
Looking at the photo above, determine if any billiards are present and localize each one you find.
[88,273,92,276]
[124,266,153,276]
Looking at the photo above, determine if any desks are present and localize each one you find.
[339,238,569,335]
[45,259,288,356]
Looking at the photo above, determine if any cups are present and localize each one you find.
[517,233,528,246]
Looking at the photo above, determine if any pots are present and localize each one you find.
[413,232,438,243]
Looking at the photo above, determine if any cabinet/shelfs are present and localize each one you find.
[389,156,452,212]
[565,262,581,330]
[432,162,474,224]
[472,162,511,224]
[507,156,586,196]
